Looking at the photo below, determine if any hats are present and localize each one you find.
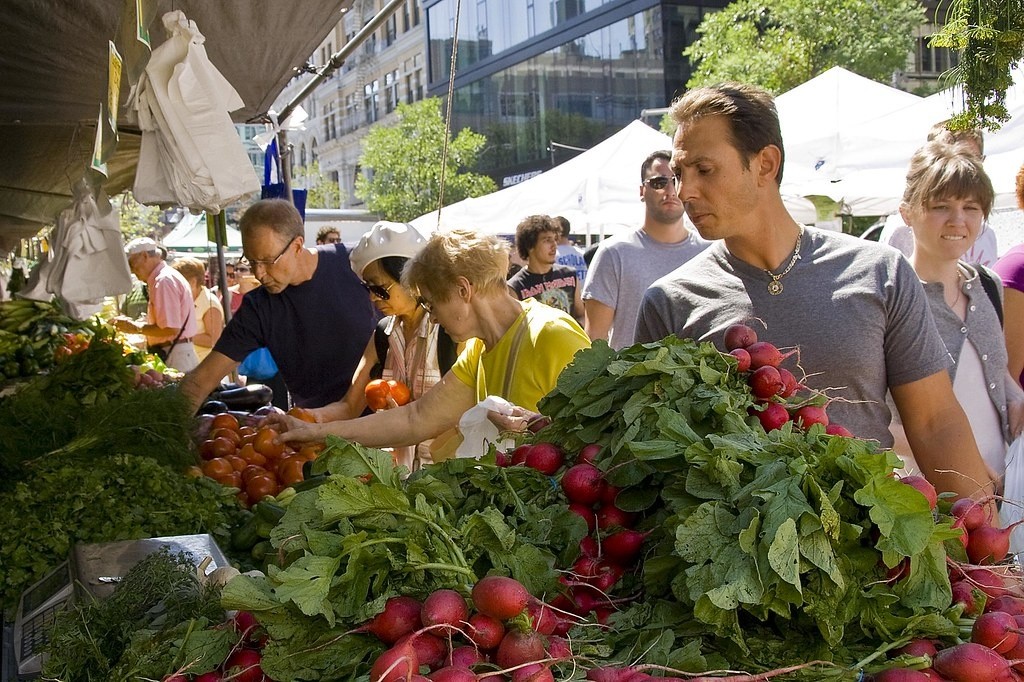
[349,220,427,282]
[124,237,156,253]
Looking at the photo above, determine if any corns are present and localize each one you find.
[0,297,81,340]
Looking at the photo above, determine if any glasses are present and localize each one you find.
[239,237,296,268]
[420,274,473,313]
[327,238,341,244]
[363,281,401,299]
[227,273,234,278]
[976,154,987,163]
[642,177,677,190]
[236,267,249,272]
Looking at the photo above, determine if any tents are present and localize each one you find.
[403,63,1024,247]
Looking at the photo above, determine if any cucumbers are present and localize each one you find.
[230,459,333,567]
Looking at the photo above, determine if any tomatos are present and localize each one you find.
[366,379,411,409]
[192,408,370,503]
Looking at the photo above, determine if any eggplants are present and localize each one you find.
[195,382,279,424]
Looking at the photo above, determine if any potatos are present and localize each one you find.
[130,365,178,391]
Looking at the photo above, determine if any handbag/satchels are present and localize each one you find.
[997,431,1024,565]
[261,136,307,225]
[148,349,166,363]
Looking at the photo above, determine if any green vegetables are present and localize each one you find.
[0,382,237,638]
[0,314,145,405]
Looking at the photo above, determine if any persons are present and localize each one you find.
[107,82,1024,534]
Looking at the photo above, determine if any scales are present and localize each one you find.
[15,533,230,674]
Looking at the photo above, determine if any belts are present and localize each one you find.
[151,338,192,348]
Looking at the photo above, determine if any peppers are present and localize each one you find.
[0,333,176,377]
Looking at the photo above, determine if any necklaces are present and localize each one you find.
[763,222,807,295]
[949,270,963,309]
